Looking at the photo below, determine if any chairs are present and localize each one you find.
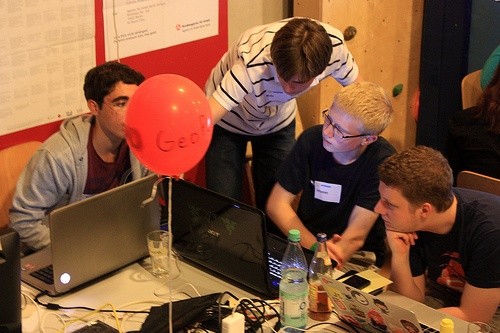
[0,141,43,228]
[461,69,483,110]
[457,171,500,196]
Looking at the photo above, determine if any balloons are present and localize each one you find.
[125,73,213,177]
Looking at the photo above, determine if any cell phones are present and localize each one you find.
[342,275,370,290]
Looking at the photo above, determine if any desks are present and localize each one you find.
[139,254,482,333]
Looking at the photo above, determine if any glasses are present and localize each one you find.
[322,109,374,139]
[100,100,128,110]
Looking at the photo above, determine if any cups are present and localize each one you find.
[467,321,491,333]
[147,230,170,277]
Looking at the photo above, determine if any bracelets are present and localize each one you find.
[309,242,320,251]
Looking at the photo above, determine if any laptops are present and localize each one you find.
[165,177,338,301]
[21,172,163,297]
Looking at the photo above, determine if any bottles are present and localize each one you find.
[440,318,454,333]
[279,229,309,333]
[309,233,332,321]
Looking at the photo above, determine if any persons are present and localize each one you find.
[375,146,500,333]
[204,16,361,235]
[266,82,397,271]
[448,45,500,187]
[9,63,157,251]
